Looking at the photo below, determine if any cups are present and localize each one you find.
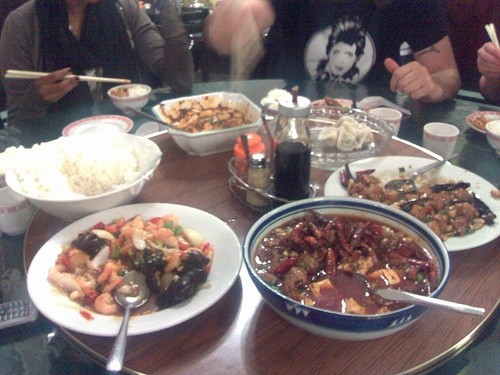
[369,108,461,161]
[0,152,34,237]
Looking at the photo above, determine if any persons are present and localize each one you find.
[477,39,500,103]
[0,0,194,127]
[203,1,462,104]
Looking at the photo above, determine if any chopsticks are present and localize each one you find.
[3,70,132,83]
[485,22,500,50]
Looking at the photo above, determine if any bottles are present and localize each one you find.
[234,94,312,210]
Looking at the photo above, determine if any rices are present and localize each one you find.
[262,88,292,109]
[126,84,148,98]
[11,124,141,201]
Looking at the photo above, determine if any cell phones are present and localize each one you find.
[0,300,38,328]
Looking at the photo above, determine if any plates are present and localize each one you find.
[62,115,133,133]
[324,156,500,253]
[312,98,358,112]
[466,111,500,133]
[27,201,242,338]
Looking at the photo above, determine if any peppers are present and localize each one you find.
[339,165,496,226]
[272,207,432,313]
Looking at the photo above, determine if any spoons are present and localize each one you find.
[106,272,151,374]
[358,96,411,116]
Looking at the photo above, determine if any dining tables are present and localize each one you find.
[139,0,217,50]
[0,79,500,375]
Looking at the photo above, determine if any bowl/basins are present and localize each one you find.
[261,106,393,170]
[154,91,263,156]
[243,196,450,340]
[6,133,162,223]
[485,121,500,155]
[107,83,152,113]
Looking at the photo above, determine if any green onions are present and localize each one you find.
[96,219,183,290]
[425,209,475,235]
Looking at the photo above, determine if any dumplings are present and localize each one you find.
[317,115,373,152]
